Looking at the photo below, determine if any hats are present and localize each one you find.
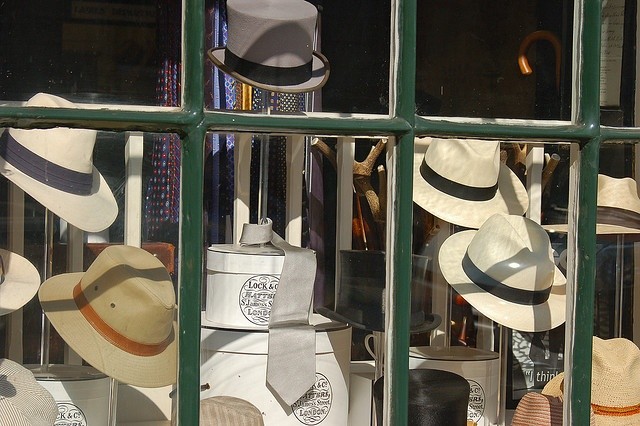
[596,174,640,234]
[1,91,119,233]
[199,396,264,426]
[540,334,640,425]
[207,0,330,93]
[511,391,595,426]
[315,250,442,334]
[412,137,529,230]
[0,358,59,426]
[38,244,178,388]
[438,213,567,334]
[371,368,470,426]
[0,248,41,316]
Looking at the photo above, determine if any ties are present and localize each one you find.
[239,217,318,407]
